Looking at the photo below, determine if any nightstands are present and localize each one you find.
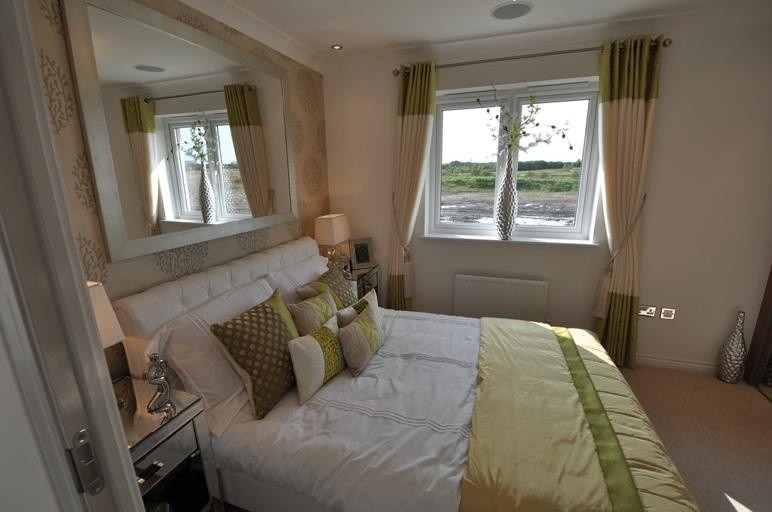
[346,262,382,306]
[110,376,226,512]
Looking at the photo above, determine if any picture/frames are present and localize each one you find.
[349,237,374,270]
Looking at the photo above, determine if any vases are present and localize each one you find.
[717,310,747,384]
[199,162,216,225]
[494,145,519,241]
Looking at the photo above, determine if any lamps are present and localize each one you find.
[86,280,128,350]
[314,213,352,260]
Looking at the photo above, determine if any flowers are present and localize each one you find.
[164,108,221,169]
[475,73,579,155]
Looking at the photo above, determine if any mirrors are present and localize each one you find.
[61,0,299,263]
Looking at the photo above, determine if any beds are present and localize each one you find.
[110,235,702,510]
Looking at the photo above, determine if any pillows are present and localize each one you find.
[295,263,358,311]
[145,277,289,436]
[285,290,338,336]
[263,254,329,295]
[210,288,302,420]
[338,303,385,378]
[337,289,379,328]
[288,315,344,404]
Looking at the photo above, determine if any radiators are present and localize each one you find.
[451,272,550,323]
